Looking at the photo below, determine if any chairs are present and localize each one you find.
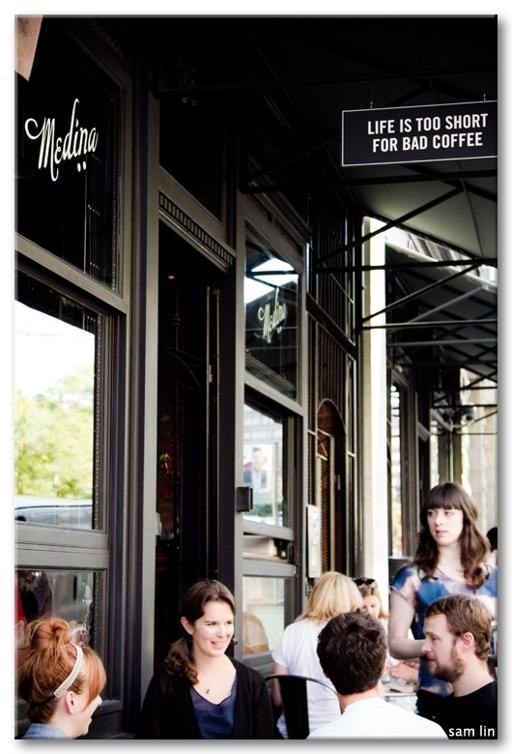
[263,674,343,738]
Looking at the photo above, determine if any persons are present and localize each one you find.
[381,479,498,695]
[269,570,497,739]
[126,575,286,739]
[18,611,107,739]
[244,444,269,490]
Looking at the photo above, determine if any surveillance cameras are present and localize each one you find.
[465,407,476,421]
